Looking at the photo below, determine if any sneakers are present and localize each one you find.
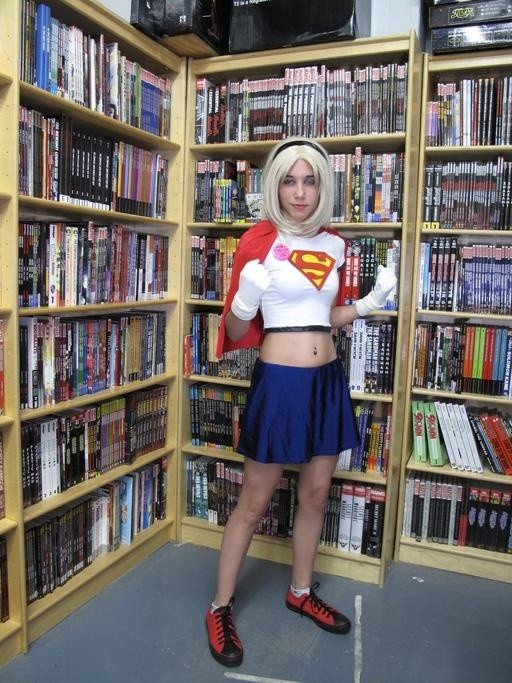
[205,602,243,668]
[285,585,351,635]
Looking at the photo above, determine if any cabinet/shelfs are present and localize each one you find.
[18,0,190,657]
[386,47,512,588]
[1,0,25,674]
[175,28,428,591]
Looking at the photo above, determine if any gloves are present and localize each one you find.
[354,260,399,318]
[229,255,272,324]
